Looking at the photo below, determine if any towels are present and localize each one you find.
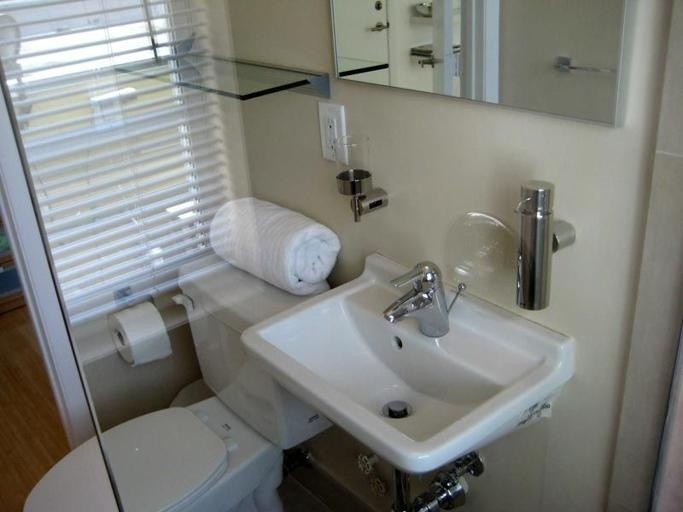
[210,195,341,297]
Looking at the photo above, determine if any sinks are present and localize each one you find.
[240,247,576,473]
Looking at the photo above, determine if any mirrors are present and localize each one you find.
[327,1,628,130]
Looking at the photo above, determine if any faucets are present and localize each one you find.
[380,258,449,337]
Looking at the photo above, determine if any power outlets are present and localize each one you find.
[315,101,348,166]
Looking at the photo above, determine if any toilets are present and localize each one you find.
[20,253,334,512]
